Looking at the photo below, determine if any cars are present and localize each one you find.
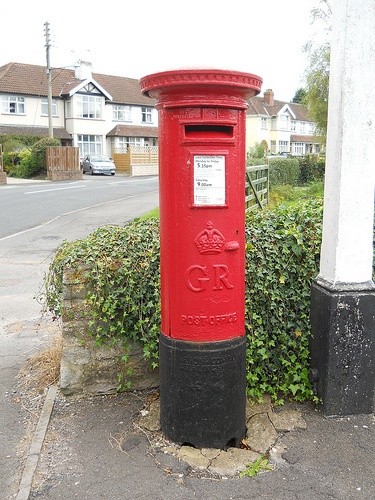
[280,152,293,157]
[82,155,116,176]
